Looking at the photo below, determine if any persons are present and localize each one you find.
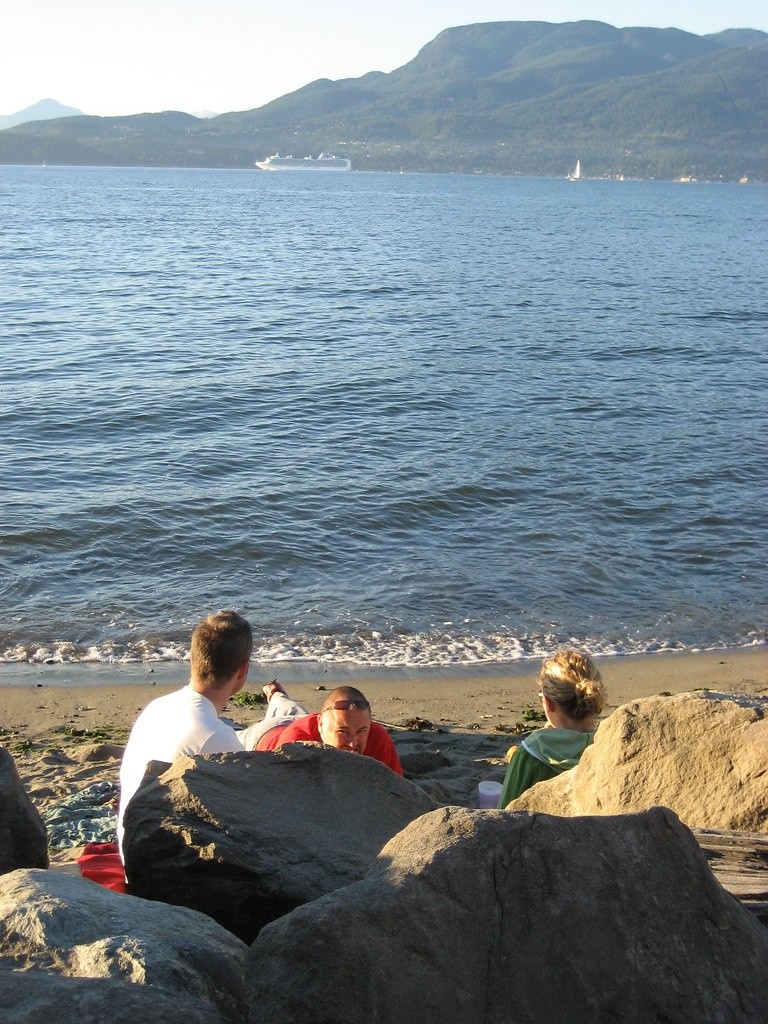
[497,650,606,809]
[118,608,253,892]
[218,678,403,779]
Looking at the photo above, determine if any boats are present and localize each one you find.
[680,178,697,183]
[739,177,751,184]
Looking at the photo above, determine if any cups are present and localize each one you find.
[478,780,504,809]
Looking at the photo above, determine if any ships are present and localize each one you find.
[254,152,351,171]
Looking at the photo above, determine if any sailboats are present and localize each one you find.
[569,159,581,182]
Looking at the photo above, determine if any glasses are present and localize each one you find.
[539,690,544,703]
[322,700,371,714]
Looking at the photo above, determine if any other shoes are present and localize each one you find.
[262,679,289,704]
